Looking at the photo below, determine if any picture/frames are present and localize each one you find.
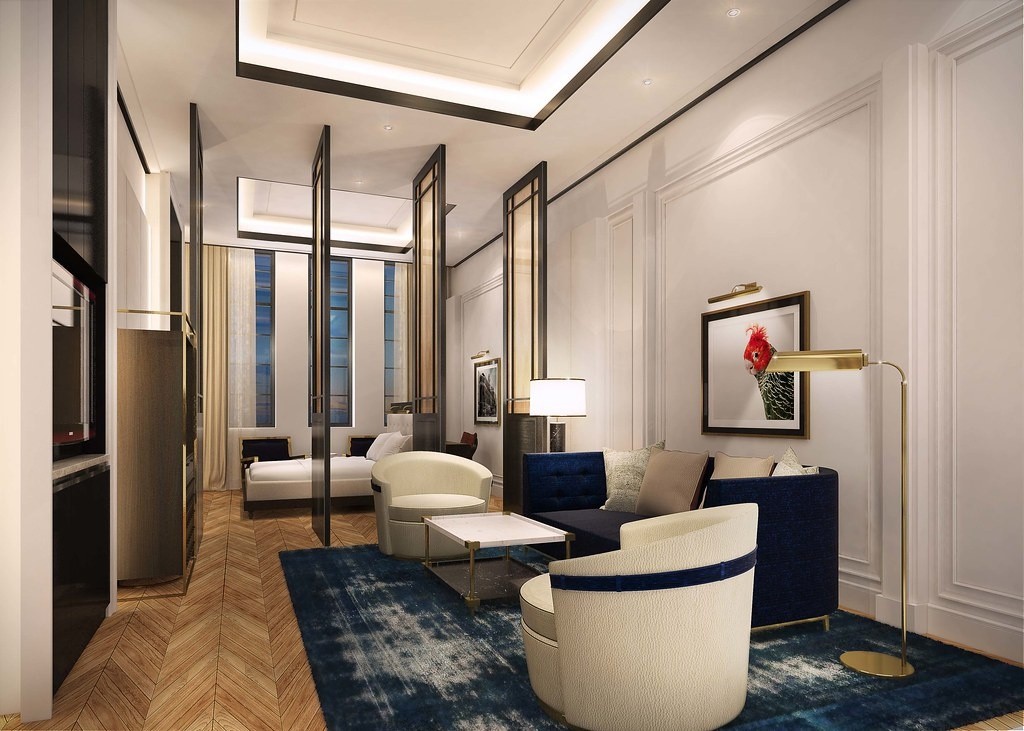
[701,288,811,440]
[472,357,502,426]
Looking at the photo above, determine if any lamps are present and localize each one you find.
[708,282,763,304]
[470,349,491,359]
[764,346,916,681]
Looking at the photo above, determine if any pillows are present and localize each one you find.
[633,448,708,517]
[696,452,775,511]
[376,434,413,460]
[771,447,821,476]
[601,439,664,513]
[365,432,394,460]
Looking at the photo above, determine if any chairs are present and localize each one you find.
[238,435,307,480]
[341,434,378,459]
[460,431,478,454]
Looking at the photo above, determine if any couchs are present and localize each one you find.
[520,503,759,731]
[522,447,839,635]
[368,451,493,560]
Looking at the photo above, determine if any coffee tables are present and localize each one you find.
[420,510,573,613]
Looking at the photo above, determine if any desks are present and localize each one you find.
[309,452,337,459]
[445,440,471,458]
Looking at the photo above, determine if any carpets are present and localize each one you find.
[270,538,1024,731]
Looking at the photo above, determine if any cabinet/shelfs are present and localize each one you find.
[114,308,199,603]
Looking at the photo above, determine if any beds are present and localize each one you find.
[246,414,413,520]
